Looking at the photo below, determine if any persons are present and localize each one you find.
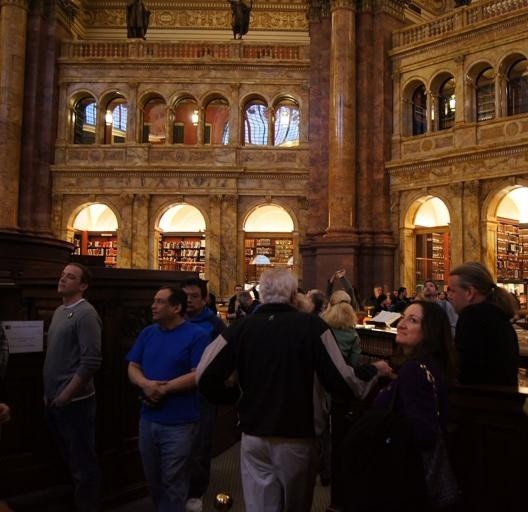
[125,284,213,511]
[196,269,391,511]
[367,302,450,510]
[180,277,226,510]
[446,262,528,509]
[227,269,459,486]
[43,261,103,510]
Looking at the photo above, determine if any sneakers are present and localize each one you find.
[186,497,203,512]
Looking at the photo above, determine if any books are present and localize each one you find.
[429,231,450,281]
[158,237,205,272]
[72,234,117,268]
[243,238,294,273]
[498,219,528,282]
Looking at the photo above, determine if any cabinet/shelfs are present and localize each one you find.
[71,234,276,284]
[422,216,528,281]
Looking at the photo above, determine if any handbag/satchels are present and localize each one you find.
[421,428,466,510]
[334,382,426,510]
